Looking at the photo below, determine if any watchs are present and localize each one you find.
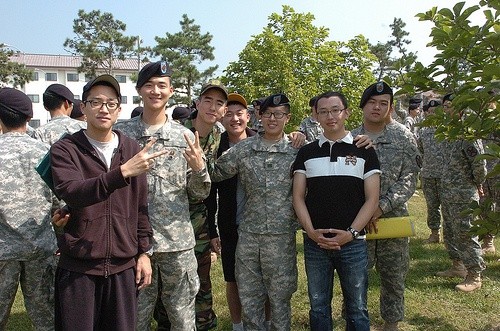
[146,247,153,256]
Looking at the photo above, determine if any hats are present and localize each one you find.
[409,98,441,112]
[83,74,121,99]
[70,99,84,118]
[359,81,393,110]
[172,106,191,119]
[473,86,496,96]
[227,92,247,109]
[252,96,269,108]
[136,60,173,88]
[47,84,76,104]
[442,93,455,104]
[259,93,291,115]
[200,80,229,100]
[1,87,34,119]
[309,97,317,107]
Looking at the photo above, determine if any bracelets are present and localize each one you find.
[348,227,359,238]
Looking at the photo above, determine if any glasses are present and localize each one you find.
[83,98,119,111]
[317,106,346,117]
[262,109,288,119]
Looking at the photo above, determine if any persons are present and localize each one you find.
[51,74,170,331]
[405,88,500,291]
[213,94,374,331]
[131,107,143,118]
[248,99,264,130]
[153,79,228,331]
[52,62,211,331]
[0,83,87,331]
[348,82,422,331]
[171,107,191,119]
[211,94,306,331]
[299,96,324,142]
[291,93,382,331]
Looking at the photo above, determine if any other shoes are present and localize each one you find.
[384,321,417,331]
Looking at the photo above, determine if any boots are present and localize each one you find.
[437,260,468,278]
[422,228,442,245]
[479,237,495,256]
[456,272,482,291]
